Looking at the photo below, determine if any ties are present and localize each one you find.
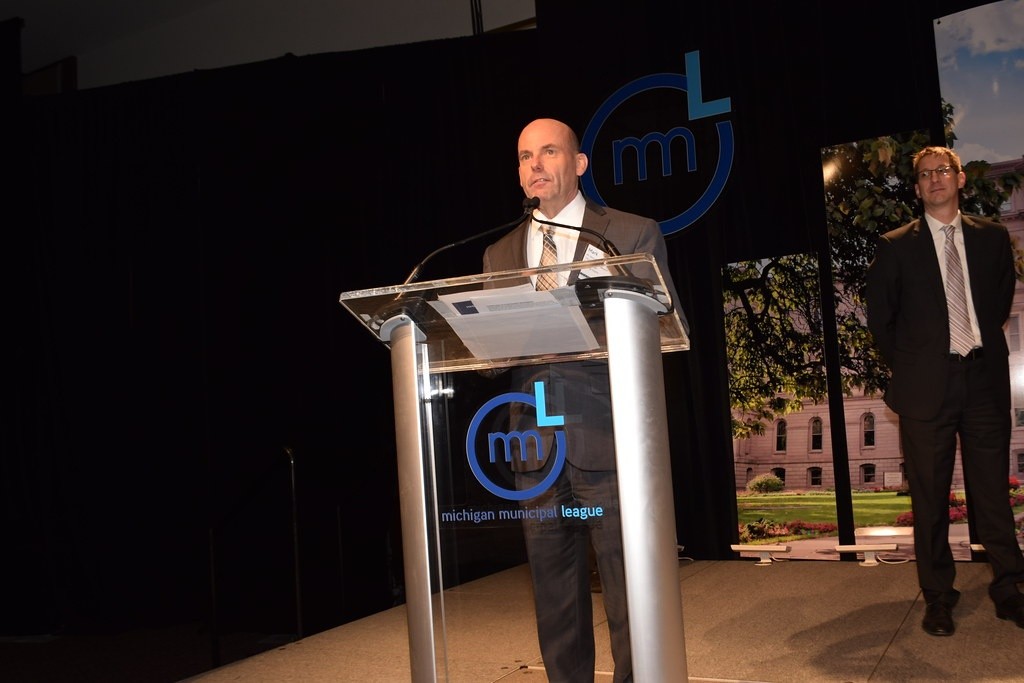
[535,225,560,292]
[940,224,975,356]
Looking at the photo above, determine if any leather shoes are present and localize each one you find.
[996,592,1024,629]
[923,602,954,635]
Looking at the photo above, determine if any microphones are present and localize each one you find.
[393,196,635,300]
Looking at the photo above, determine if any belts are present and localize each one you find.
[946,349,983,362]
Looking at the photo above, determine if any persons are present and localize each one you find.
[472,117,691,683]
[863,144,1024,638]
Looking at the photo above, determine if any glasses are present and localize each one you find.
[914,165,953,178]
